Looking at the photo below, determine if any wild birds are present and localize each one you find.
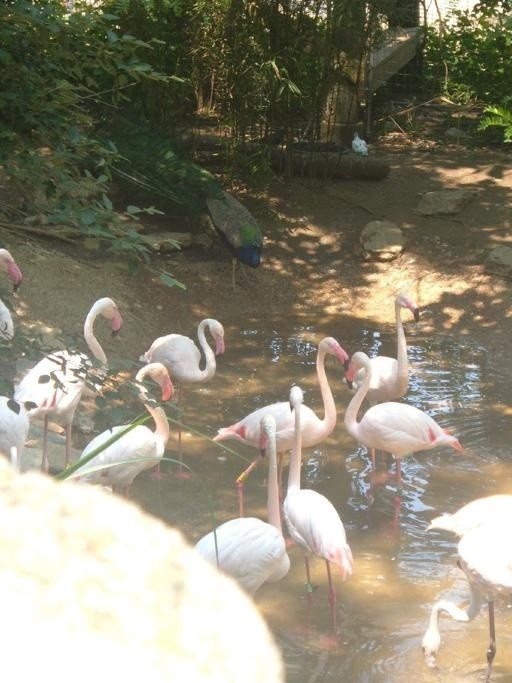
[348,130,370,158]
[1,396,31,469]
[64,361,175,497]
[15,294,125,467]
[190,290,469,645]
[201,187,264,286]
[138,316,228,386]
[422,485,510,682]
[1,246,27,342]
